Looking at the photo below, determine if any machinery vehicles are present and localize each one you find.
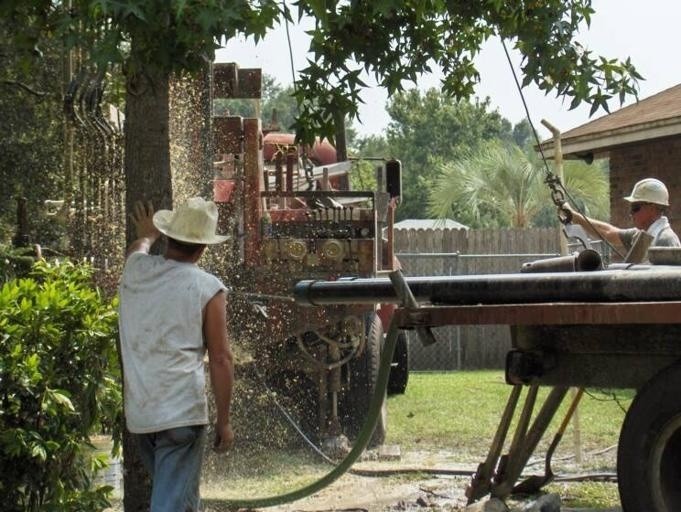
[168,58,413,452]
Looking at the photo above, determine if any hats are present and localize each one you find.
[623,177,670,206]
[152,197,231,246]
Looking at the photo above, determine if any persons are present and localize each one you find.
[117,196,236,507]
[557,177,680,265]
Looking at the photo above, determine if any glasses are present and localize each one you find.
[632,204,650,213]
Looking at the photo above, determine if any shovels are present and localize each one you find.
[510,388,586,494]
